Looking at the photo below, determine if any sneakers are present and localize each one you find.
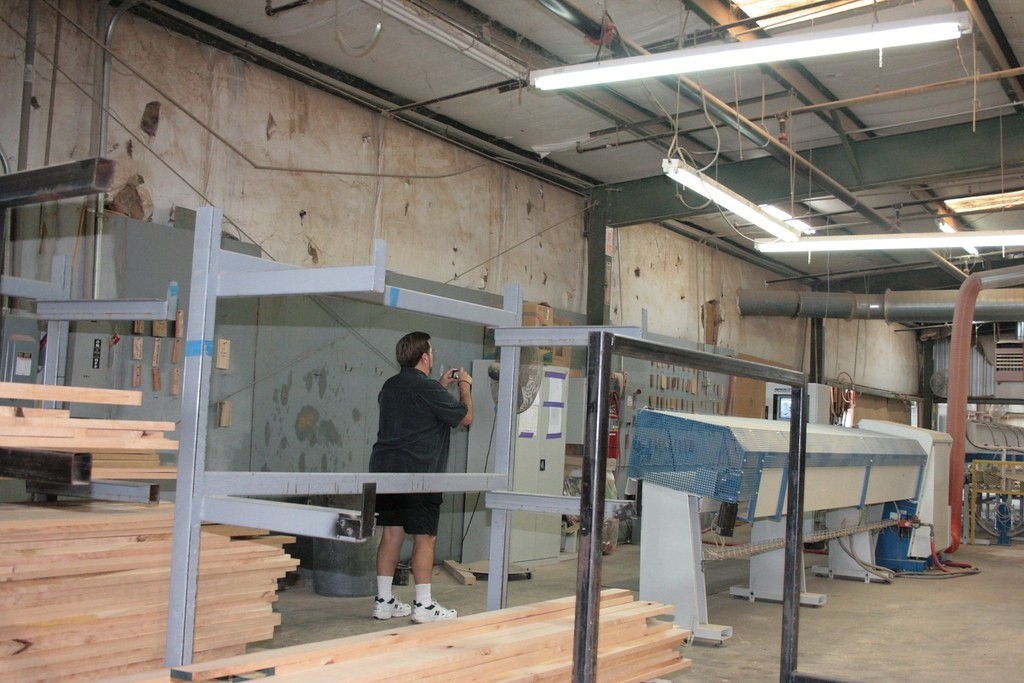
[373,595,412,620]
[411,599,457,624]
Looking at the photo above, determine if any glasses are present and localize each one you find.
[429,350,437,357]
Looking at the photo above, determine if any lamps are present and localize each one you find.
[527,0,973,91]
[753,112,1024,253]
[662,5,803,243]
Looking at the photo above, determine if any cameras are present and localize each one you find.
[452,370,460,380]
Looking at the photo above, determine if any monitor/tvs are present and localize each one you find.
[773,394,792,420]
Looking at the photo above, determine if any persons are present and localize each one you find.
[369,331,473,622]
